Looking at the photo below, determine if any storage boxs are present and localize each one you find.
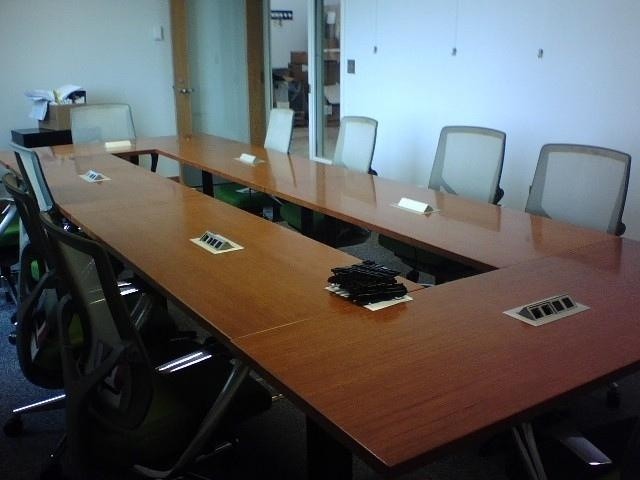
[38,102,88,132]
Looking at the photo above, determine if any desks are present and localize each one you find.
[264,130,625,273]
[229,239,640,480]
[10,128,72,148]
[156,143,362,193]
[9,152,212,218]
[0,132,246,166]
[69,190,425,479]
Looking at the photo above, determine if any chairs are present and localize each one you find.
[7,139,80,345]
[524,143,632,236]
[1,171,153,480]
[2,211,274,479]
[279,115,377,249]
[0,180,20,325]
[511,407,639,480]
[69,102,159,173]
[214,107,296,210]
[378,124,506,277]
